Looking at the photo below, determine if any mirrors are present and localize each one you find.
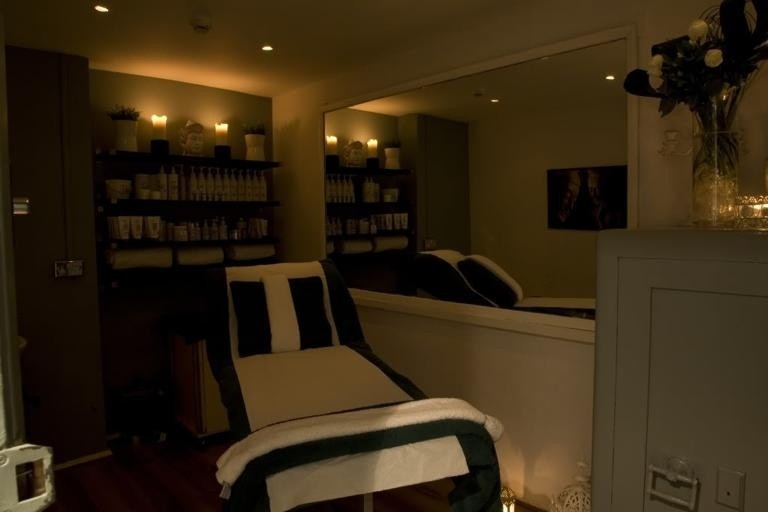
[319,24,638,345]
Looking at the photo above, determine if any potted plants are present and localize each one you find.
[242,125,267,161]
[109,107,137,152]
[384,142,400,169]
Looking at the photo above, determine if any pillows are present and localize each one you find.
[230,280,333,356]
[459,252,523,308]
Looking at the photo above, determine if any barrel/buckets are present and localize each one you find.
[382,186,400,202]
[102,179,131,200]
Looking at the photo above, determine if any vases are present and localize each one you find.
[692,132,739,227]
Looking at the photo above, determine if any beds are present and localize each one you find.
[417,247,596,319]
[205,258,523,512]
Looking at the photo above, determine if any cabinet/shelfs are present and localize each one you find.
[94,147,285,307]
[591,226,767,511]
[326,154,417,266]
[174,336,228,438]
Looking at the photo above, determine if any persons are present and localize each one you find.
[342,139,363,168]
[587,169,627,230]
[549,169,582,224]
[178,119,205,158]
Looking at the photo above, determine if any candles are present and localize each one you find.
[151,115,167,140]
[215,122,228,145]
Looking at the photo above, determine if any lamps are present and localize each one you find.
[500,487,517,512]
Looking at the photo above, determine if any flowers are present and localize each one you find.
[624,0,768,177]
